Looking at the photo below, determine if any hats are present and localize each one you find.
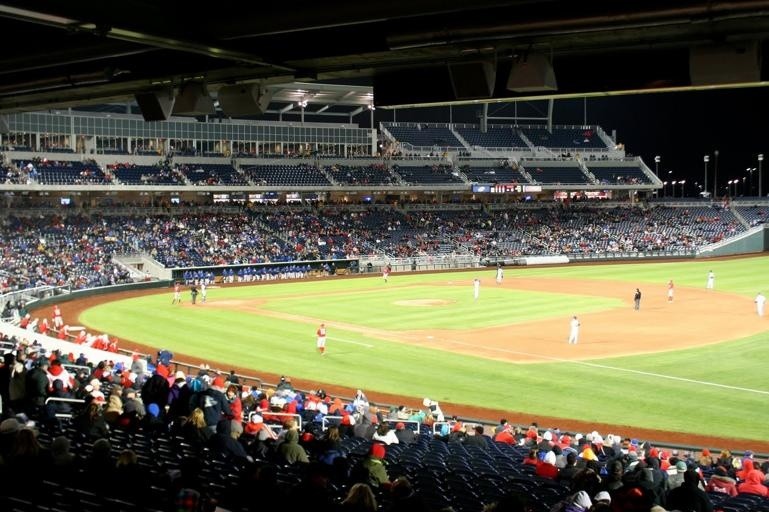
[0,416,25,435]
[745,448,752,457]
[676,460,687,472]
[631,438,638,446]
[40,357,52,368]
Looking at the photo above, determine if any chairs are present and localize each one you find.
[0,117,768,293]
[2,333,769,510]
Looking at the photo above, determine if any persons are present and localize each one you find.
[667,280,673,303]
[169,281,181,305]
[1,153,768,290]
[189,282,197,304]
[754,292,765,317]
[1,304,769,508]
[200,281,206,302]
[470,278,480,303]
[633,288,640,310]
